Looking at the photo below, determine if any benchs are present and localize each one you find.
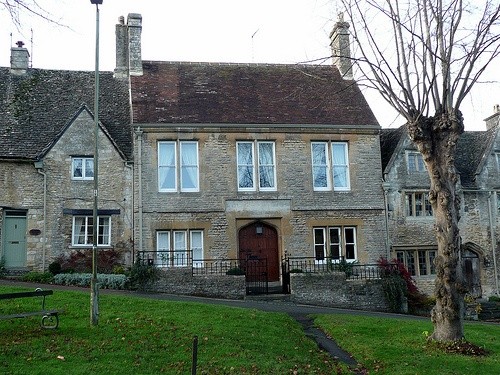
[0,288,62,330]
